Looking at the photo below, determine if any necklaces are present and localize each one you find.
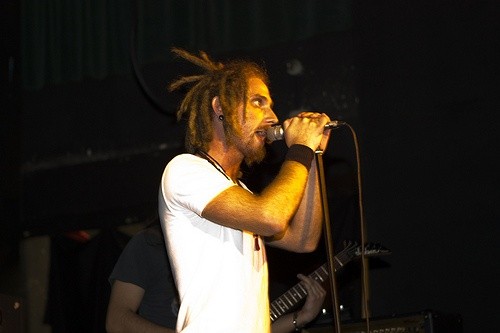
[195,147,261,251]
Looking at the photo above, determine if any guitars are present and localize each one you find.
[270,239,392,324]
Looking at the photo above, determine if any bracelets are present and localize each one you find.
[293,311,300,331]
[284,144,315,171]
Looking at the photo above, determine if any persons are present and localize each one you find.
[157,47,332,333]
[105,214,327,333]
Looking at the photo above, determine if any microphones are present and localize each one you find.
[268,120,346,140]
